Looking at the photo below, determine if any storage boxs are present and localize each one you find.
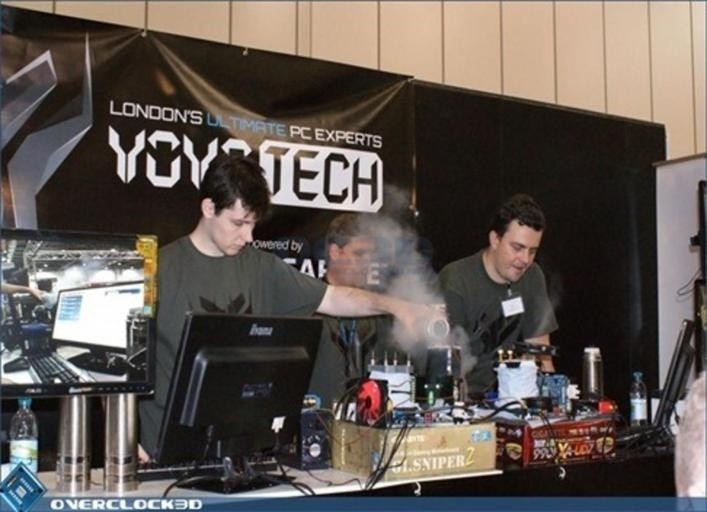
[496,418,617,470]
[330,422,496,480]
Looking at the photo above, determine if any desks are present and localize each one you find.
[0,452,672,506]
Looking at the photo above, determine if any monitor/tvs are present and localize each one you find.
[651,319,697,455]
[154,310,324,494]
[51,279,145,375]
[0,226,160,399]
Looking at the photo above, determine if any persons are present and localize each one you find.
[423,192,560,393]
[308,214,408,418]
[0,283,48,302]
[101,152,442,465]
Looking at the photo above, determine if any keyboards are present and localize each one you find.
[614,425,662,446]
[136,455,278,482]
[24,350,97,386]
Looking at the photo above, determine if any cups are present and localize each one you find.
[101,391,143,495]
[412,317,454,341]
[55,395,90,494]
[427,344,463,383]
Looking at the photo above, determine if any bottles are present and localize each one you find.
[7,398,40,477]
[628,372,650,428]
[581,345,604,401]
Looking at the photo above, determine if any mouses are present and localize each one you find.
[4,358,29,373]
[90,468,105,485]
